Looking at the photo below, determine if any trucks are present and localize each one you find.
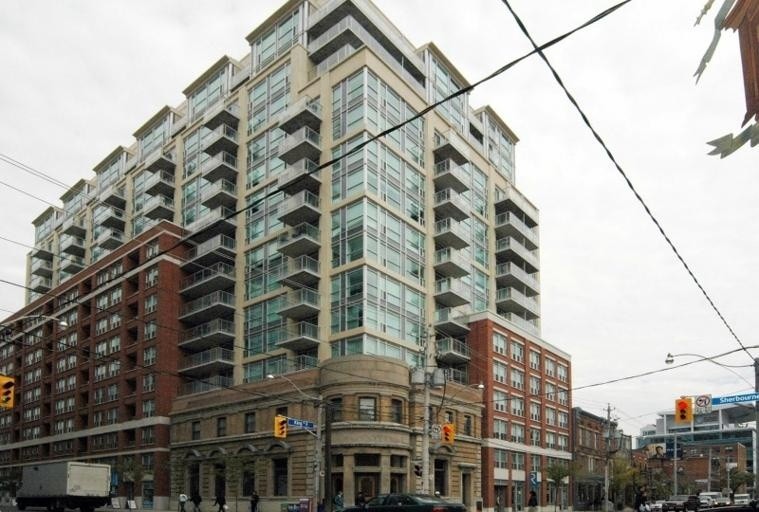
[15,460,112,512]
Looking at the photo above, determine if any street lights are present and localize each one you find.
[0,314,68,333]
[264,372,324,512]
[442,381,485,408]
[665,353,758,502]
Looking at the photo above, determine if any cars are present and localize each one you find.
[342,494,468,512]
[639,492,759,512]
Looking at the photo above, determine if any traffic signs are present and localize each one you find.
[710,392,759,407]
[287,418,314,429]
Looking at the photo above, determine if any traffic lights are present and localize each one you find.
[678,401,688,421]
[0,376,15,409]
[274,417,286,438]
[442,424,454,443]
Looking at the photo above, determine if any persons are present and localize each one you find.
[355,492,365,504]
[212,490,226,512]
[528,490,539,512]
[633,485,653,512]
[497,494,504,511]
[333,491,344,512]
[651,445,667,458]
[250,490,260,512]
[188,490,202,512]
[178,491,189,512]
